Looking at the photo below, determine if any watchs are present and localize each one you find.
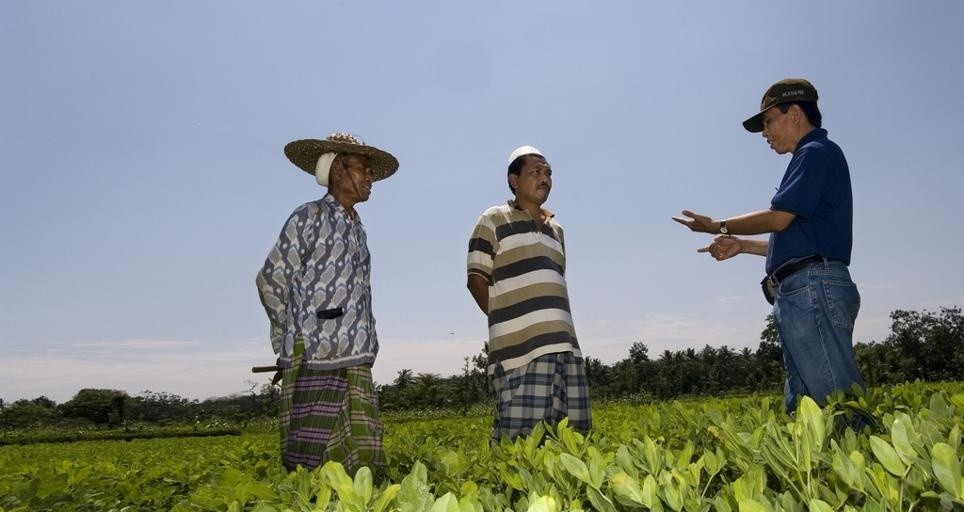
[719,219,728,235]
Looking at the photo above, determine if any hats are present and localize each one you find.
[507,144,547,176]
[284,130,401,183]
[743,77,820,132]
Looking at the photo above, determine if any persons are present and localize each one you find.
[462,142,597,448]
[256,126,402,493]
[670,78,867,415]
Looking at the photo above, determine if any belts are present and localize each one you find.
[766,254,841,287]
